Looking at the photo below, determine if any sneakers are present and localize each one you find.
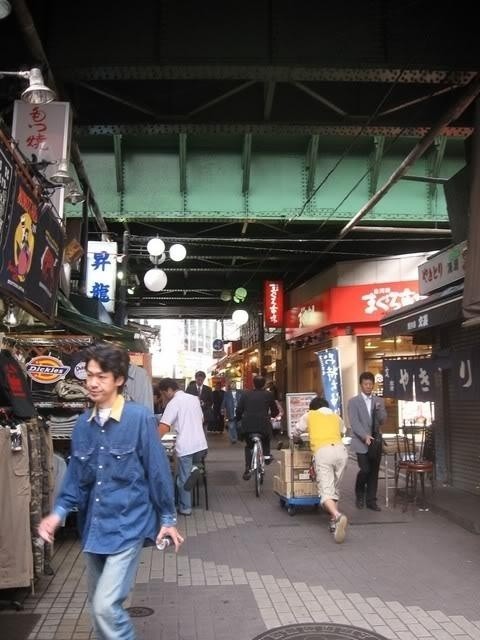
[356,495,364,510]
[334,514,348,544]
[183,469,200,492]
[206,429,245,444]
[264,455,275,465]
[177,509,192,516]
[242,467,253,481]
[329,518,337,532]
[366,500,380,512]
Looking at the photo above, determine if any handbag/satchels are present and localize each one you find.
[368,406,383,460]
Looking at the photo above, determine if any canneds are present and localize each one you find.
[155,537,174,550]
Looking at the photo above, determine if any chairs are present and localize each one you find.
[175,448,211,511]
[394,417,437,518]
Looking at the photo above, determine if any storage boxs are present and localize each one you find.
[271,448,320,498]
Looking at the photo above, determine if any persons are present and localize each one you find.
[235,375,280,480]
[293,395,347,543]
[347,371,388,511]
[211,381,226,433]
[185,371,213,442]
[17,227,29,274]
[35,348,183,640]
[222,383,240,444]
[155,376,208,515]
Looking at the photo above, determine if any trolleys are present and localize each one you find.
[273,440,324,515]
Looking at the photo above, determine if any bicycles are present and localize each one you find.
[242,433,270,498]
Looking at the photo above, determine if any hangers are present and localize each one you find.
[0,335,91,364]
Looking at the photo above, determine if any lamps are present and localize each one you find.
[142,231,186,292]
[0,67,88,208]
